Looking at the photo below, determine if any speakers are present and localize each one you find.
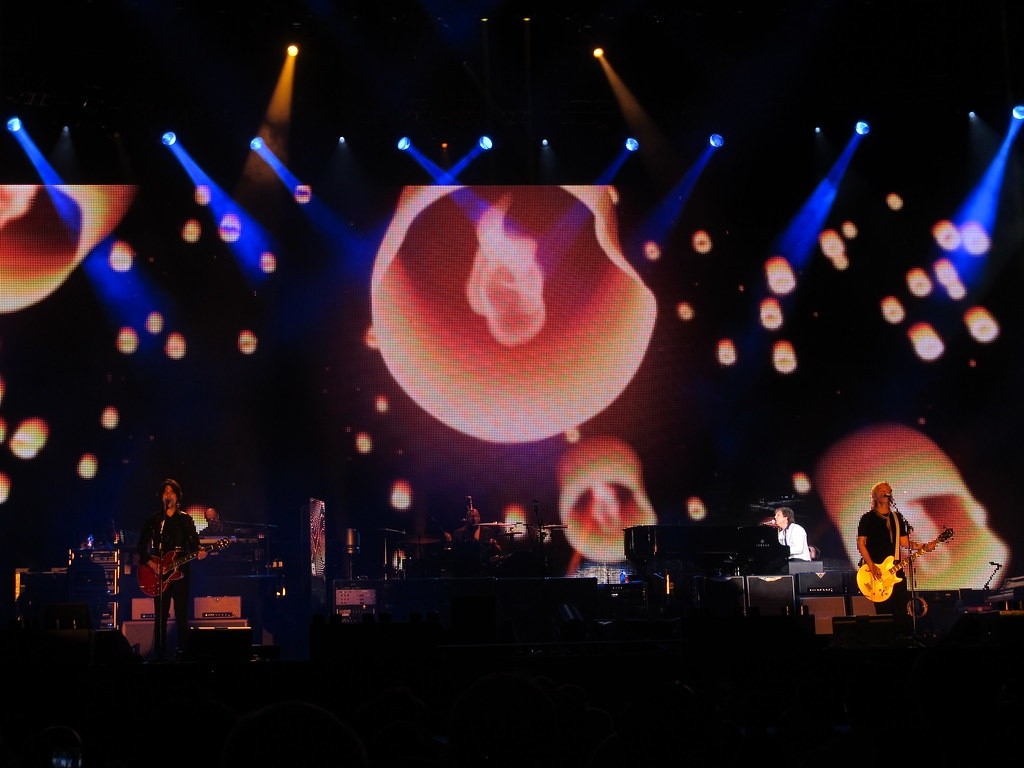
[44,620,251,670]
[683,573,960,648]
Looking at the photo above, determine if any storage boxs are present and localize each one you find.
[747,574,796,617]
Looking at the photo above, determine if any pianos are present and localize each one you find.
[621,524,791,579]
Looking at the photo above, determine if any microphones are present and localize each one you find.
[533,499,538,512]
[989,562,1002,567]
[883,494,891,498]
[165,498,172,510]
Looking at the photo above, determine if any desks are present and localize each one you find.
[800,597,877,634]
[123,619,248,658]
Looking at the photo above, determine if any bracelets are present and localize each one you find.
[867,562,873,566]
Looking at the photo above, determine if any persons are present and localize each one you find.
[200,508,235,536]
[858,482,937,629]
[135,477,208,663]
[774,507,812,574]
[443,508,502,552]
[0,625,1024,768]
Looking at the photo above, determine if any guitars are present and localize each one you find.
[856,525,955,603]
[137,537,229,598]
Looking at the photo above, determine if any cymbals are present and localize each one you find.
[537,524,568,529]
[373,527,406,536]
[473,521,516,526]
[497,531,523,536]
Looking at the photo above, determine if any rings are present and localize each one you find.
[877,572,880,575]
